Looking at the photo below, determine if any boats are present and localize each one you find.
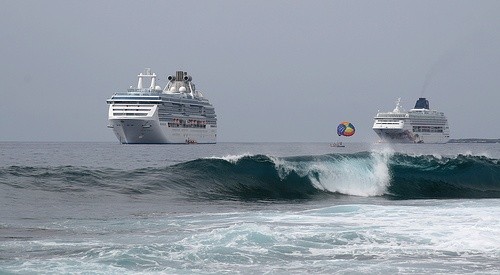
[328,142,345,148]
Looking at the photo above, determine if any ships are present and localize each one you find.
[371,97,450,145]
[104,69,218,145]
